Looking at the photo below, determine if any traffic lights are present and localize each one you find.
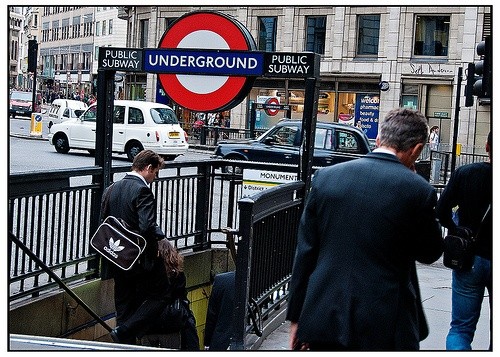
[474,34,493,98]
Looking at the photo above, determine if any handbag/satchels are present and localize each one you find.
[443,227,474,269]
[90,215,147,271]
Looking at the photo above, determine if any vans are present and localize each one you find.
[47,99,92,132]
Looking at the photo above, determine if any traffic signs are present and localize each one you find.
[241,169,298,201]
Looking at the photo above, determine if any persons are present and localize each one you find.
[37,89,96,112]
[437,132,492,350]
[285,106,444,350]
[118,92,125,99]
[347,120,367,148]
[191,110,230,146]
[426,125,439,161]
[203,271,235,350]
[276,127,302,148]
[98,150,173,344]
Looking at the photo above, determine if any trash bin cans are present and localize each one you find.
[31,113,43,137]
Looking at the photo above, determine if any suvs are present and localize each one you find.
[210,118,371,184]
[48,100,189,162]
[10,91,42,118]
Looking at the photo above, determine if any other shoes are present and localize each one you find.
[111,324,136,344]
